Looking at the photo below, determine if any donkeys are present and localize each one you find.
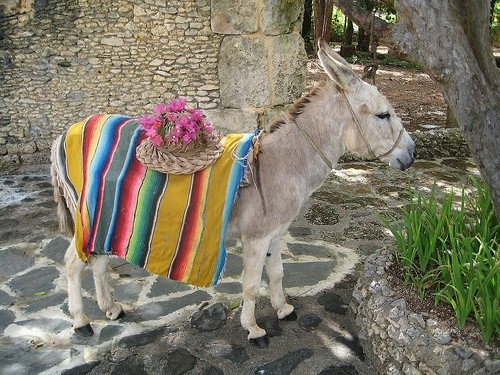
[48,36,418,349]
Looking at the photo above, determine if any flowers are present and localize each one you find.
[137,98,215,147]
